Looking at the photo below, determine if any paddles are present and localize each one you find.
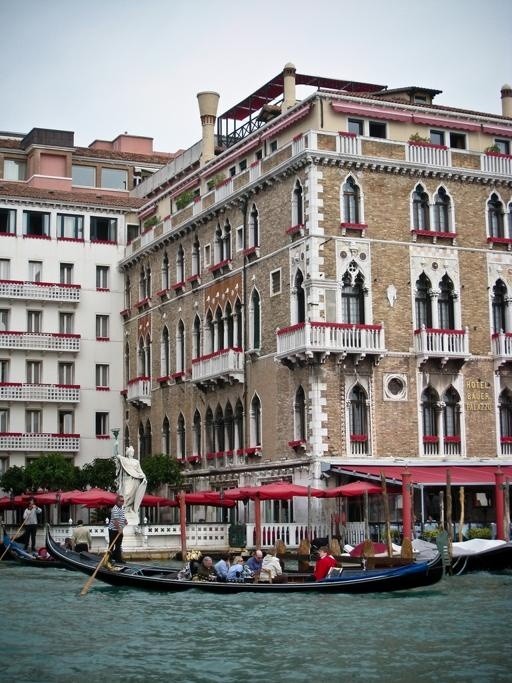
[79,518,127,595]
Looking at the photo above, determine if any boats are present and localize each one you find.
[44,522,444,594]
[343,533,512,574]
[2,522,70,566]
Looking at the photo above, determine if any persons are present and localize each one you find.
[114,445,148,513]
[424,515,438,524]
[108,495,128,561]
[73,518,92,552]
[23,502,43,552]
[60,537,73,552]
[197,546,336,583]
